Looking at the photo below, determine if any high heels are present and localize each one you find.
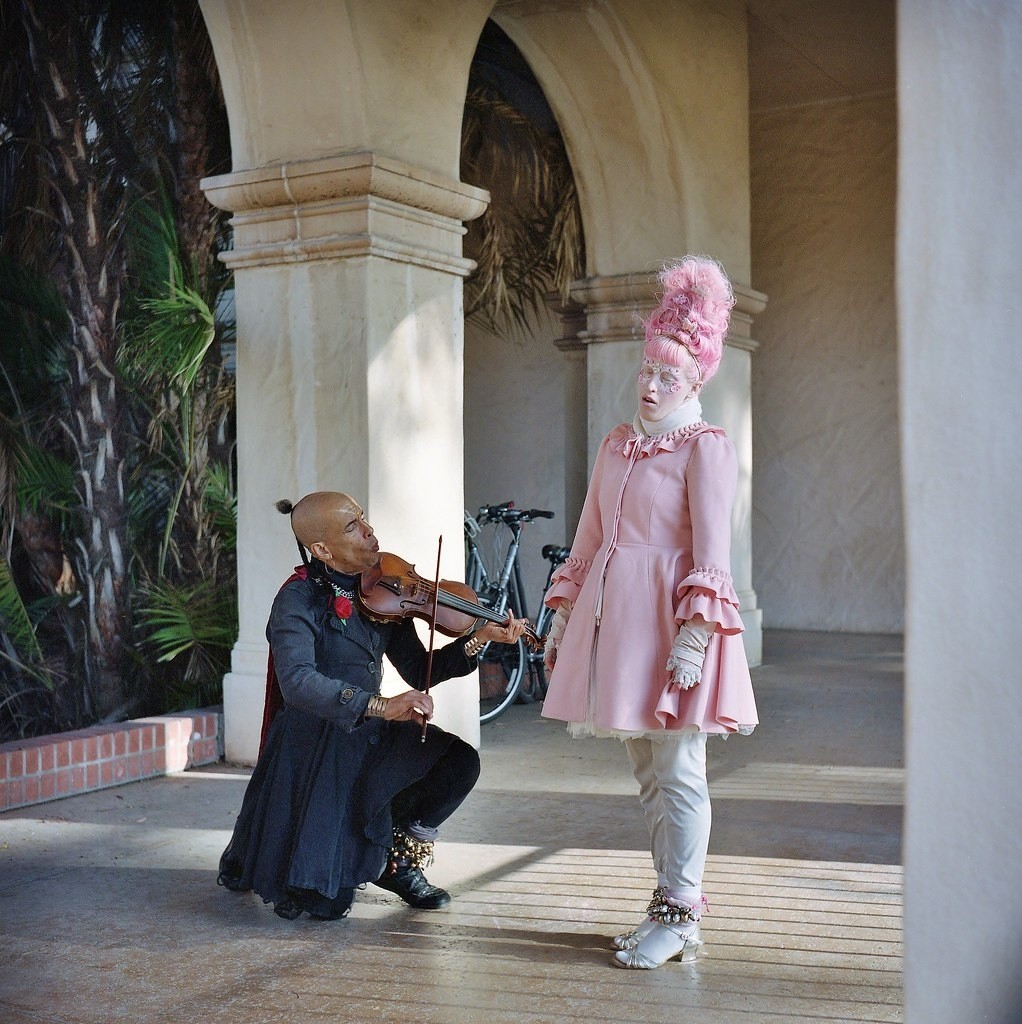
[609,914,703,971]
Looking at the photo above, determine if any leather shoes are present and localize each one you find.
[369,832,452,910]
[218,813,253,891]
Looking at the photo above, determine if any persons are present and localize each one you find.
[542,253,760,969]
[218,491,531,922]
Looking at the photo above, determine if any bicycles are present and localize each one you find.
[462,500,572,725]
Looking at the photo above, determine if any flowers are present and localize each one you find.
[334,590,354,626]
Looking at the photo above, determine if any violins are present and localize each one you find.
[353,552,550,652]
